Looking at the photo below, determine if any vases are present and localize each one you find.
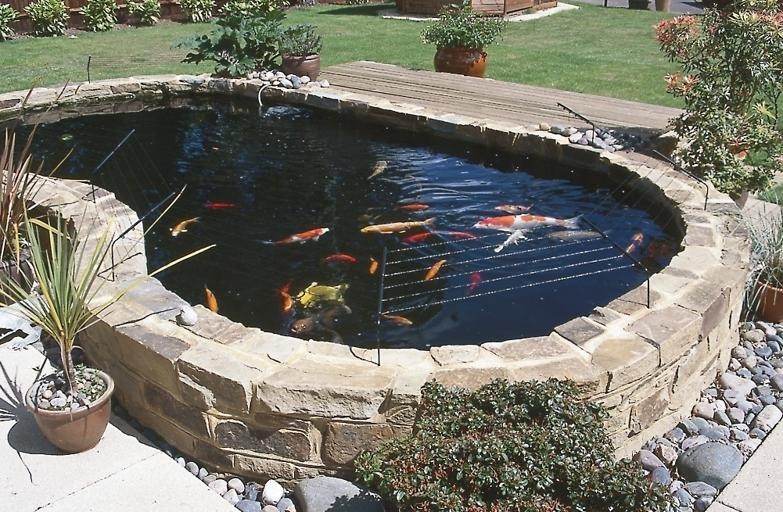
[727,189,751,210]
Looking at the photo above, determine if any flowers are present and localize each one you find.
[650,0,782,193]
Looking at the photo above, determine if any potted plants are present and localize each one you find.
[727,182,783,327]
[0,183,218,454]
[0,79,136,309]
[275,22,323,82]
[419,0,511,78]
